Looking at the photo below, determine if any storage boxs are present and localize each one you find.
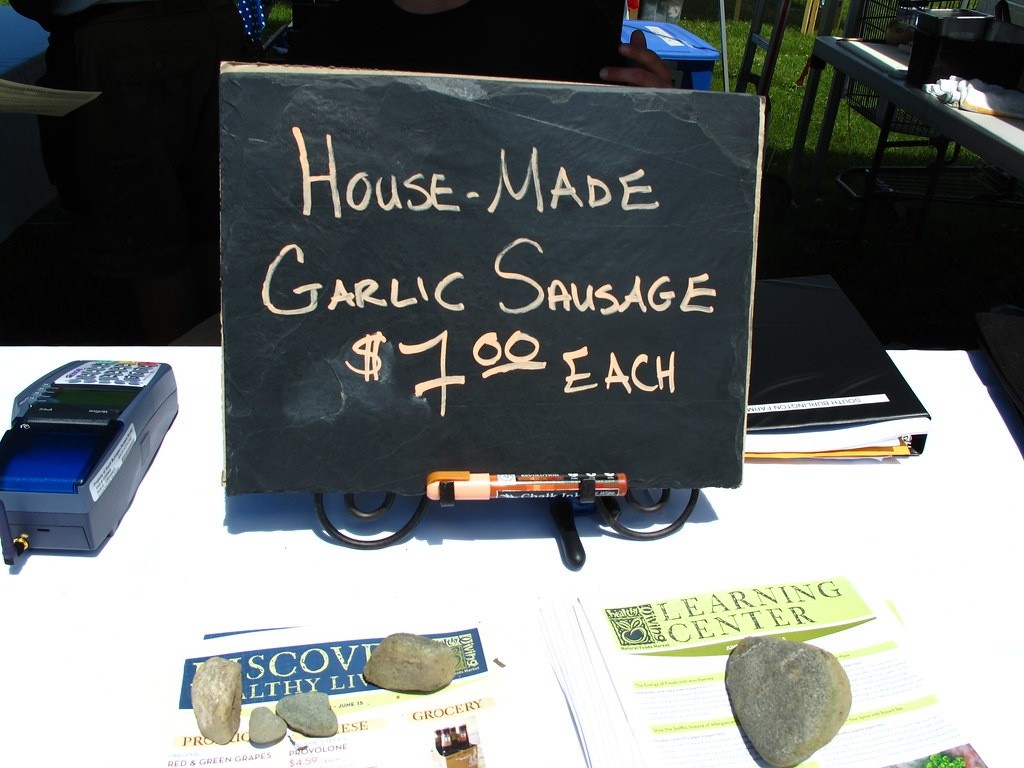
[906,29,1024,90]
[622,19,721,91]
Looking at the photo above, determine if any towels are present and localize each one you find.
[922,74,1024,120]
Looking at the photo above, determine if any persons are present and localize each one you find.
[13,0,249,346]
[283,0,676,90]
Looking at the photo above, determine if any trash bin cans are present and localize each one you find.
[620,20,721,92]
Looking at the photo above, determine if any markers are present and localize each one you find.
[424,468,628,501]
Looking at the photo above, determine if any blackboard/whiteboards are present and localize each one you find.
[218,60,767,494]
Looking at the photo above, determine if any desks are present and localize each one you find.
[807,36,1024,278]
[0,341,1024,768]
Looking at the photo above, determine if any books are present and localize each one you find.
[745,274,932,461]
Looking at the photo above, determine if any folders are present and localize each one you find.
[743,275,932,461]
